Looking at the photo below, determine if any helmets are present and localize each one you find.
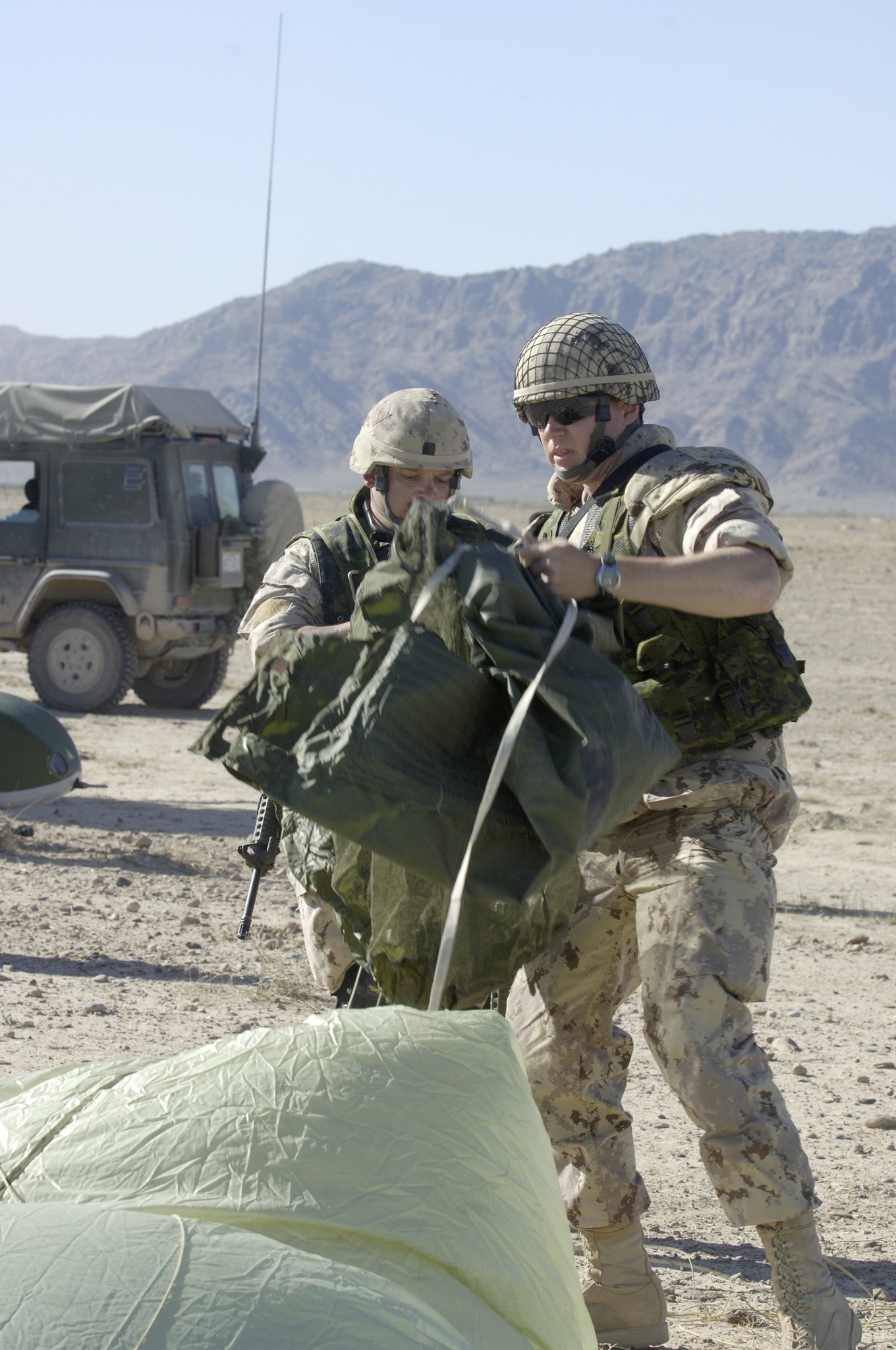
[350,387,473,480]
[513,312,660,424]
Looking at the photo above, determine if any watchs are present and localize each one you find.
[596,554,623,603]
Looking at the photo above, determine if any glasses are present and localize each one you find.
[523,396,598,429]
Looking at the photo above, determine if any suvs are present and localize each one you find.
[0,383,265,712]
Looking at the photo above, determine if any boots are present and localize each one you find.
[755,1201,862,1350]
[579,1220,670,1346]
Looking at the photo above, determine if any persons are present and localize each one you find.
[184,473,211,524]
[256,389,473,1014]
[510,310,867,1350]
[6,478,42,525]
